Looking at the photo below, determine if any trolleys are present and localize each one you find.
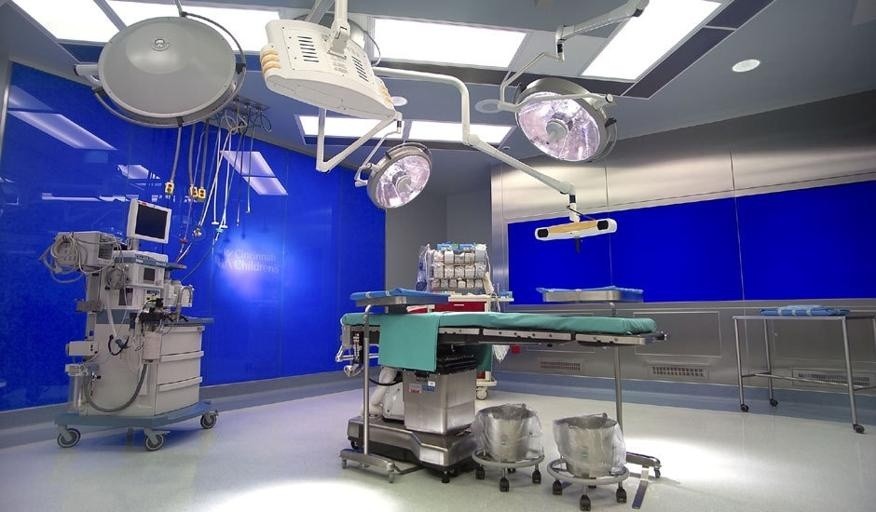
[56,402,218,451]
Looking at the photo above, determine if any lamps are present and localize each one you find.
[355,115,431,210]
[516,79,618,165]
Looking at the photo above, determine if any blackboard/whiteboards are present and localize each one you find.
[504,169,875,307]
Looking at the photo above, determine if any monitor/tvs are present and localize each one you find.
[126,199,172,243]
[57,231,116,267]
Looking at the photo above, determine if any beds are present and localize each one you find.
[337,313,670,484]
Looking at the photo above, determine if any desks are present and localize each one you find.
[731,307,875,434]
[351,290,516,401]
